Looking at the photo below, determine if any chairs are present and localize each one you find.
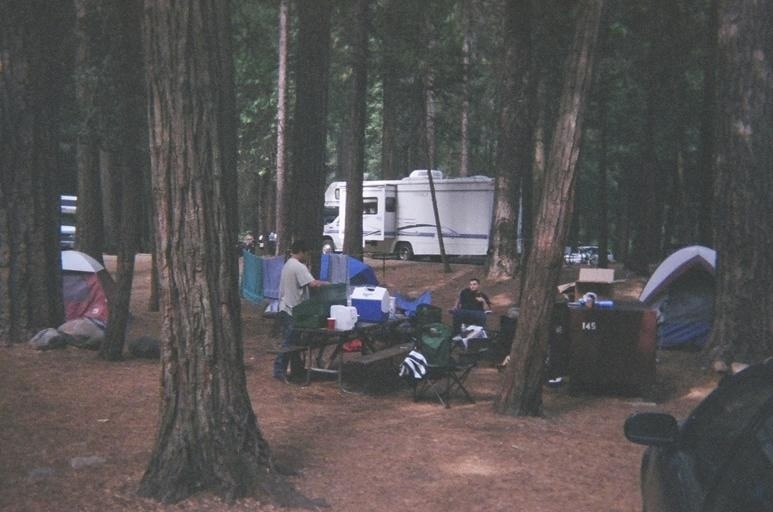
[403,317,475,410]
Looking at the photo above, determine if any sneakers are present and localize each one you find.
[273,369,306,385]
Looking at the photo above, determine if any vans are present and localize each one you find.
[324,169,523,261]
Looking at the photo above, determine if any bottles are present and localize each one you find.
[461,323,467,338]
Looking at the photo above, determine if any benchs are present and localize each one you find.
[277,335,359,390]
[349,342,426,398]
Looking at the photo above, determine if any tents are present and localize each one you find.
[60,249,132,329]
[320,251,379,285]
[641,243,717,350]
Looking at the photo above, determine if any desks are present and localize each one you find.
[275,304,415,398]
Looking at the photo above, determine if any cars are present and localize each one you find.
[564,243,613,265]
[60,194,77,250]
[624,357,773,511]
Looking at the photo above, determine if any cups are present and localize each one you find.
[326,317,336,331]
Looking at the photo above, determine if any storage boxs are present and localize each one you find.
[557,269,627,302]
[349,286,396,322]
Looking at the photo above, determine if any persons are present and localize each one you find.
[453,278,492,334]
[273,243,332,384]
[243,230,276,252]
